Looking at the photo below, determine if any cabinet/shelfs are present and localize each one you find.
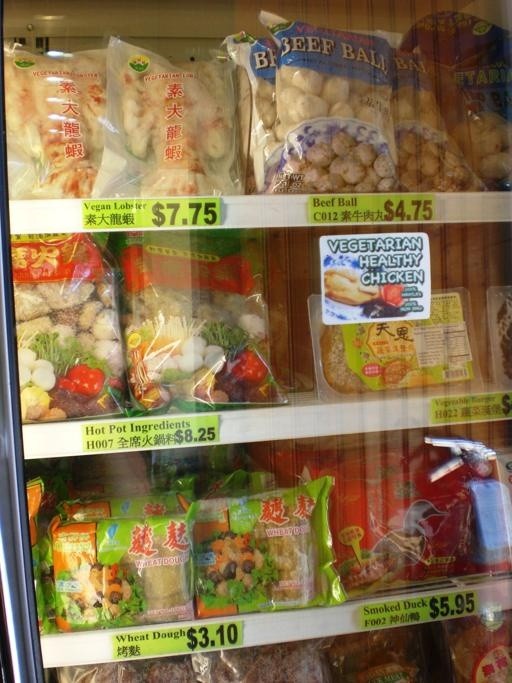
[7,189,511,669]
